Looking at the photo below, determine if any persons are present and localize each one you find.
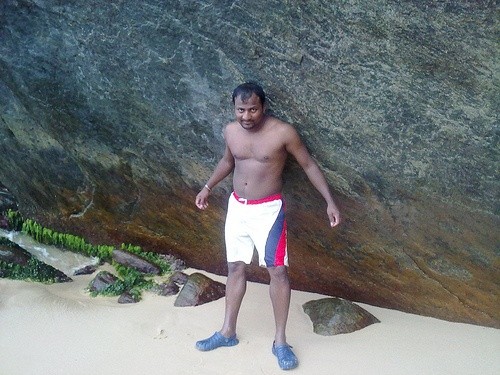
[194,82,341,371]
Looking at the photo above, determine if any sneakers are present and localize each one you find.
[196,331,239,352]
[272,340,299,370]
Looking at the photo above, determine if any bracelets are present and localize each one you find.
[204,184,212,191]
